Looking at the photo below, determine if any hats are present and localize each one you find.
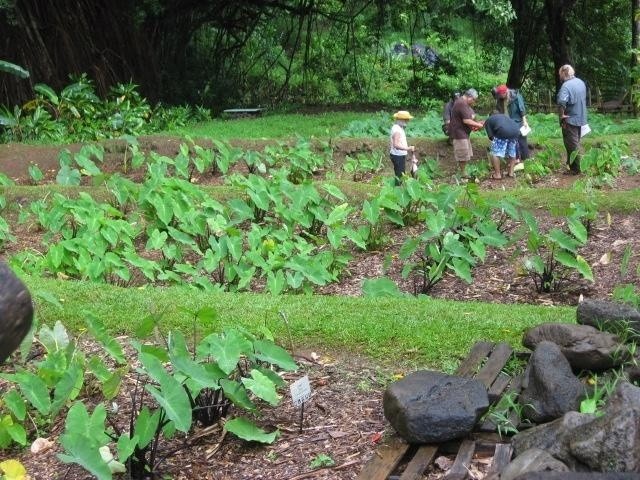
[496,85,508,94]
[393,110,414,120]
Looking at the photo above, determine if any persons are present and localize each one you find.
[389,111,418,186]
[557,64,588,175]
[442,84,530,178]
